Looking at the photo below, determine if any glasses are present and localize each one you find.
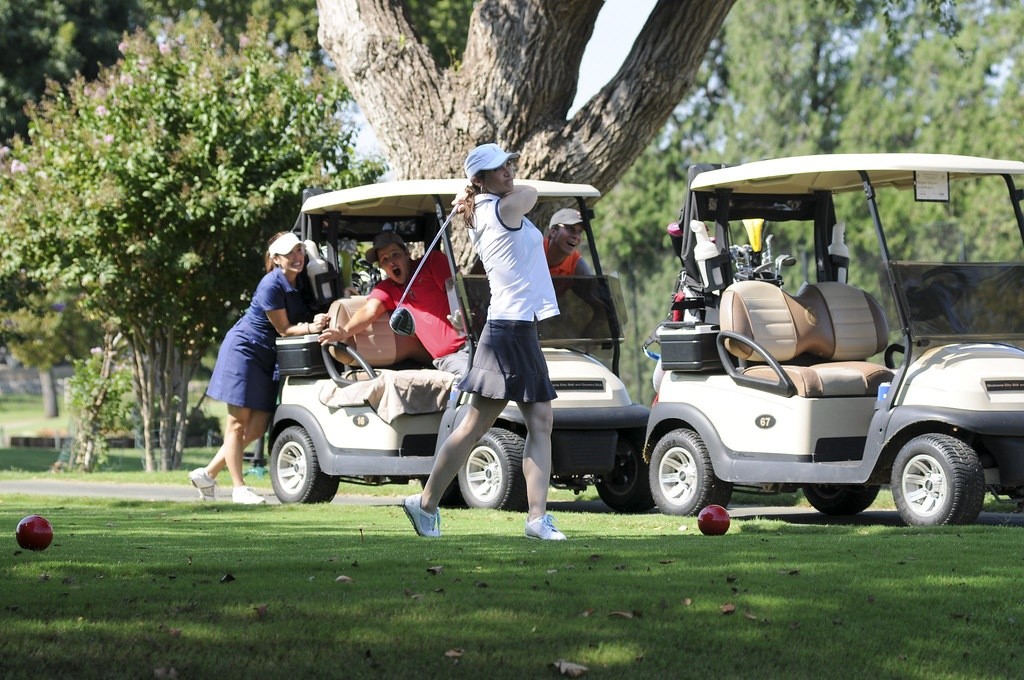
[558,222,584,234]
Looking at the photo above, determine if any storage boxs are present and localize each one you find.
[658,323,733,373]
[276,334,341,377]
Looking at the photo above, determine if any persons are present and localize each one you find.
[319,232,470,378]
[401,144,567,541]
[190,230,329,504]
[540,207,589,277]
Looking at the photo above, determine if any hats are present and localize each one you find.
[464,143,522,182]
[548,207,583,229]
[373,232,408,251]
[269,233,306,259]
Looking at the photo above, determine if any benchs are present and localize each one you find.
[715,279,894,398]
[320,294,456,407]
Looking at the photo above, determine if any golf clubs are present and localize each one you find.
[388,204,456,337]
[729,233,797,282]
[351,259,382,294]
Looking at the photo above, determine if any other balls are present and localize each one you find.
[15,514,55,551]
[695,503,732,535]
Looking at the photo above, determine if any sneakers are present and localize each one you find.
[232,485,267,506]
[524,513,567,541]
[403,493,443,538]
[189,467,219,501]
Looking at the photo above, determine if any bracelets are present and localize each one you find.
[308,323,311,333]
